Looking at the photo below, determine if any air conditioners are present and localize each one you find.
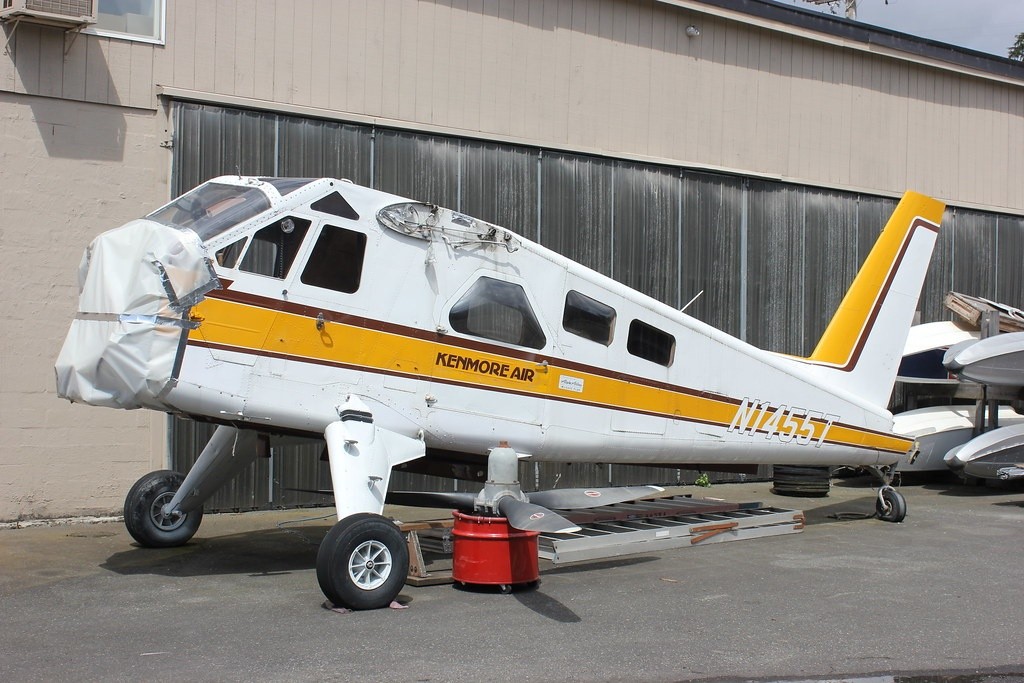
[0,0,98,29]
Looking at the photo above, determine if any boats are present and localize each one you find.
[940,329,1024,392]
[900,318,991,388]
[944,421,1024,482]
[885,403,1024,474]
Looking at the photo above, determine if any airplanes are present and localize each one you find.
[52,175,949,615]
